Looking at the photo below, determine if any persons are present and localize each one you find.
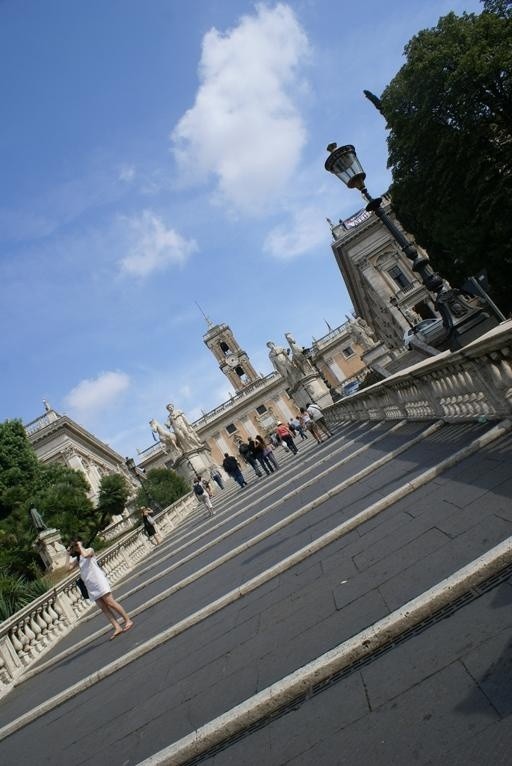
[191,402,335,517]
[67,536,134,641]
[165,403,203,454]
[267,341,298,390]
[139,506,165,546]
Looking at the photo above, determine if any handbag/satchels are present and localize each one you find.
[76,578,89,599]
[146,516,156,525]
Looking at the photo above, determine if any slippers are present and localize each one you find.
[123,621,134,632]
[109,629,123,641]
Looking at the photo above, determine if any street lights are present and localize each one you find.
[182,452,200,480]
[321,140,488,337]
[301,347,344,404]
[389,296,418,338]
[124,456,163,516]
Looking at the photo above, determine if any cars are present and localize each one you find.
[401,318,442,351]
[341,380,361,397]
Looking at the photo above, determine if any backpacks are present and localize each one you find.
[193,484,203,495]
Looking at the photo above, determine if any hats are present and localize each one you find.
[276,420,282,426]
[139,505,146,513]
[194,478,199,483]
